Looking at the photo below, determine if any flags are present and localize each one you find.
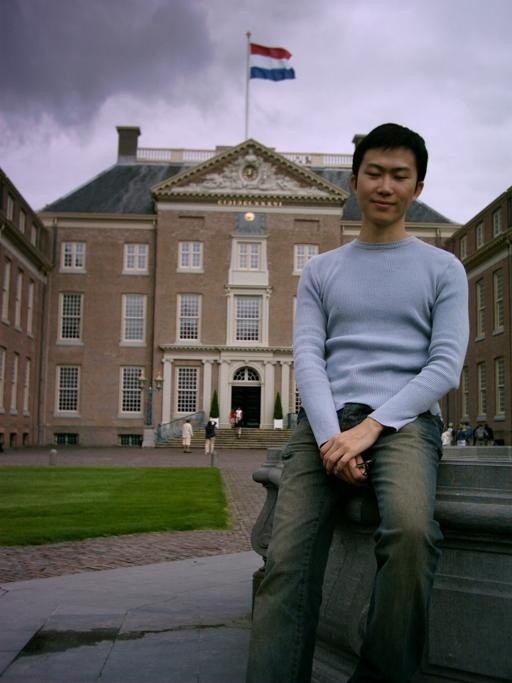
[248,42,296,82]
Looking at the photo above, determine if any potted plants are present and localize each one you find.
[273,391,284,430]
[208,389,220,429]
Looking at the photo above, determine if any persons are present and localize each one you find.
[441,421,494,447]
[204,421,217,455]
[229,406,243,439]
[181,420,193,453]
[246,122,470,683]
